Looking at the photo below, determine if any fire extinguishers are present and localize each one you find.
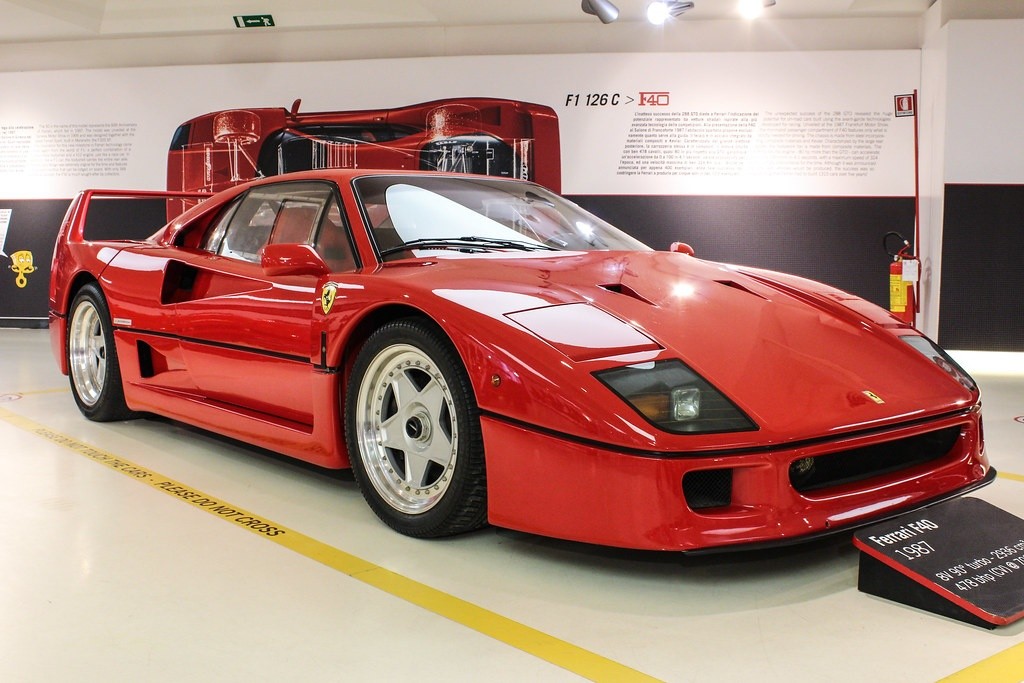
[883,231,921,330]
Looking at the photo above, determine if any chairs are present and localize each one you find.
[232,206,416,272]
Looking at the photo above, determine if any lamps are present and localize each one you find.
[582,0,619,24]
[734,0,777,19]
[646,0,694,25]
